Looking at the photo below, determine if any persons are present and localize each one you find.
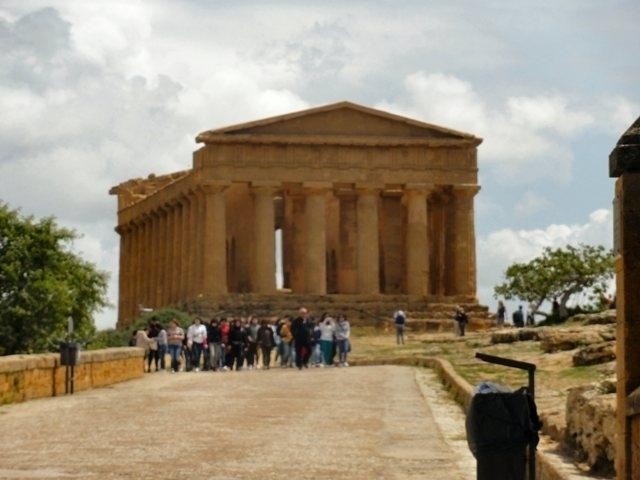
[511,304,524,328]
[451,304,471,337]
[128,307,352,374]
[393,307,408,345]
[526,310,536,327]
[495,299,508,332]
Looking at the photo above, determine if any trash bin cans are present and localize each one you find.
[474,392,531,480]
